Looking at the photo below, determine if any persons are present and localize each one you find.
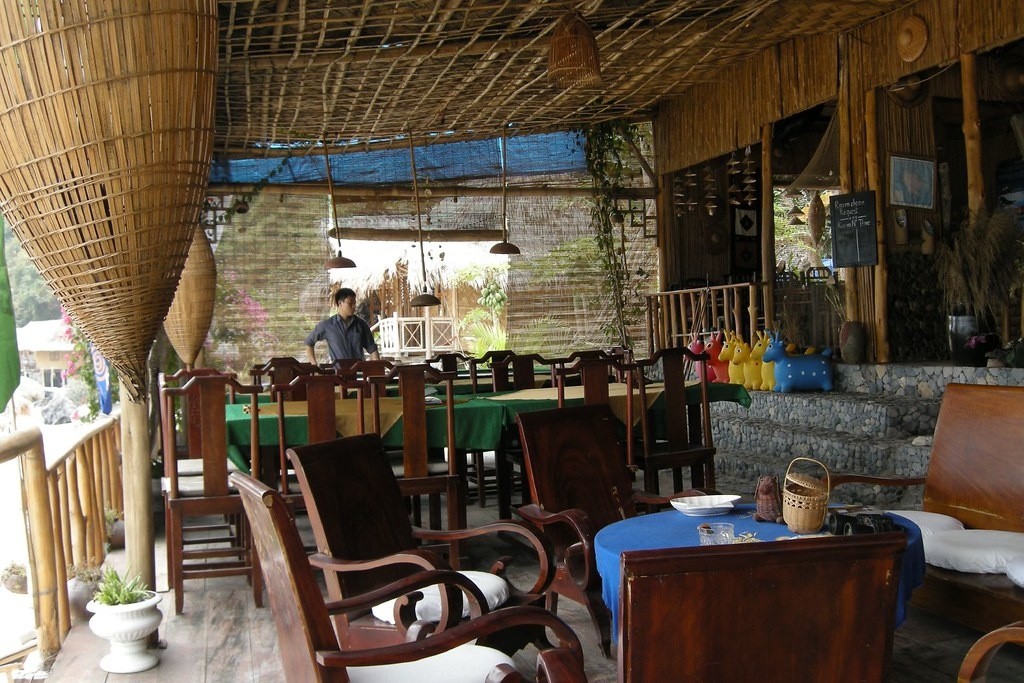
[304,288,380,366]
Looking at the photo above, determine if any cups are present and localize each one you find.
[828,513,857,535]
[843,522,875,535]
[697,522,734,546]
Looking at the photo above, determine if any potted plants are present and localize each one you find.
[105,503,124,548]
[86,566,164,675]
[3,562,26,593]
[67,558,104,620]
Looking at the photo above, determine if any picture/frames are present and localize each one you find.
[885,151,939,213]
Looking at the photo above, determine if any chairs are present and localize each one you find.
[509,397,708,657]
[619,531,905,682]
[228,470,588,682]
[153,344,718,615]
[815,382,1024,648]
[671,265,850,361]
[286,433,558,656]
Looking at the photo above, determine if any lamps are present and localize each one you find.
[323,135,356,271]
[489,125,521,253]
[409,132,442,307]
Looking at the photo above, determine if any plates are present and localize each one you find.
[669,495,742,517]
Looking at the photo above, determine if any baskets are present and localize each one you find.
[782,457,830,535]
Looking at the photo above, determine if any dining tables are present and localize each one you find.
[226,382,430,404]
[457,365,551,374]
[224,394,505,585]
[466,378,743,539]
[437,373,615,508]
[595,503,925,637]
